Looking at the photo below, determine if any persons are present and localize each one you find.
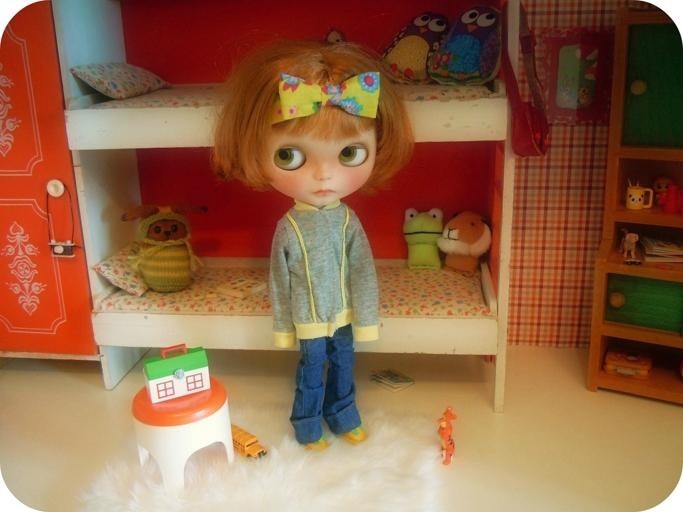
[211,29,415,446]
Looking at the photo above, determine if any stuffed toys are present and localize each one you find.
[122,200,209,294]
[438,209,493,275]
[429,7,502,88]
[379,12,446,85]
[401,206,445,272]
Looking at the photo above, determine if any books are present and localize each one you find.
[639,234,683,263]
[369,366,414,394]
